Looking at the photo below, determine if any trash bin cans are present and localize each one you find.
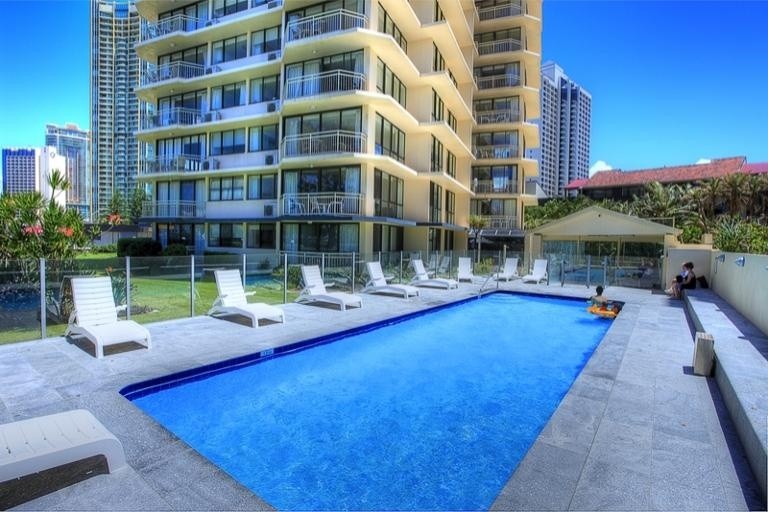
[692,332,715,376]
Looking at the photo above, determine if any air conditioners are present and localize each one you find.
[200,157,218,170]
[263,202,278,217]
[205,65,221,75]
[267,0,282,10]
[266,50,281,61]
[203,110,221,122]
[204,19,220,27]
[263,150,278,165]
[266,100,280,114]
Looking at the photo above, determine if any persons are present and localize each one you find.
[668,262,696,301]
[588,286,609,303]
[664,262,689,295]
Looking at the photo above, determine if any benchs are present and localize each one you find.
[0,408,126,479]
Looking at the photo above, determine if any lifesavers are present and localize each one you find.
[587,304,616,319]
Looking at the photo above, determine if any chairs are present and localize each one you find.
[288,195,344,213]
[64,275,151,359]
[205,269,286,328]
[294,264,363,312]
[493,257,549,285]
[361,256,475,298]
